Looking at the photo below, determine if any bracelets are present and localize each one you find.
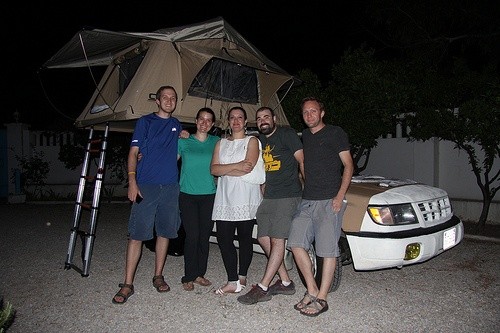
[127,171,136,175]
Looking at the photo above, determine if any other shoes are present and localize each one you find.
[183,280,193,290]
[193,277,209,286]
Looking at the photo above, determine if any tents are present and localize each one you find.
[40,18,298,133]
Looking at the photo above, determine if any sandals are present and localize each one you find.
[214,279,246,295]
[294,290,317,310]
[112,284,135,304]
[153,276,170,293]
[299,297,328,317]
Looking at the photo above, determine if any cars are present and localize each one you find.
[145,129,464,295]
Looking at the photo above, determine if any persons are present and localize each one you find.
[210,106,264,295]
[112,86,189,305]
[138,107,221,293]
[286,97,354,317]
[238,106,304,305]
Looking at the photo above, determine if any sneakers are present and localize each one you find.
[237,283,272,305]
[269,279,296,295]
